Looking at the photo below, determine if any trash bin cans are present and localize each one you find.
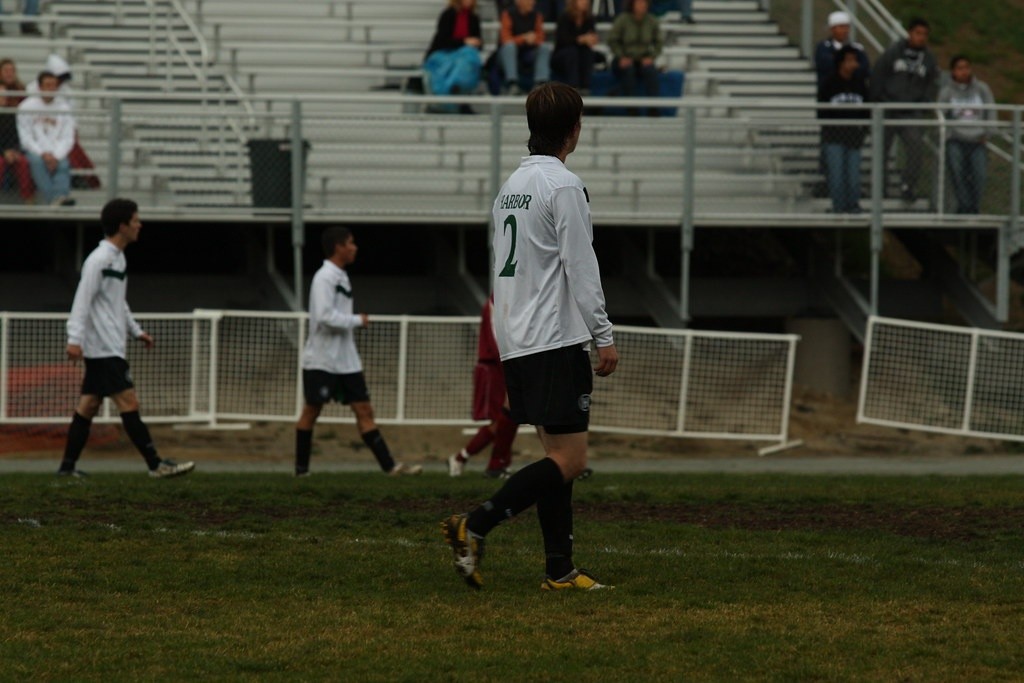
[249,138,310,208]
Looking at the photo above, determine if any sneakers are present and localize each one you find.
[541,566,616,591]
[442,513,485,589]
[447,454,463,477]
[148,458,195,480]
[388,463,421,476]
[485,469,511,479]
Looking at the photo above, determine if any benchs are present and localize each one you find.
[169,1,818,215]
[0,0,180,211]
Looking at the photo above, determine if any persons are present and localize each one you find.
[444,290,520,479]
[863,14,941,203]
[0,56,78,206]
[440,79,621,591]
[934,52,999,216]
[0,0,43,37]
[551,0,607,88]
[54,199,196,480]
[291,231,422,480]
[424,0,483,113]
[607,0,663,117]
[498,0,552,96]
[814,10,872,212]
[495,0,697,25]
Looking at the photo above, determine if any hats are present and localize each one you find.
[829,12,850,29]
